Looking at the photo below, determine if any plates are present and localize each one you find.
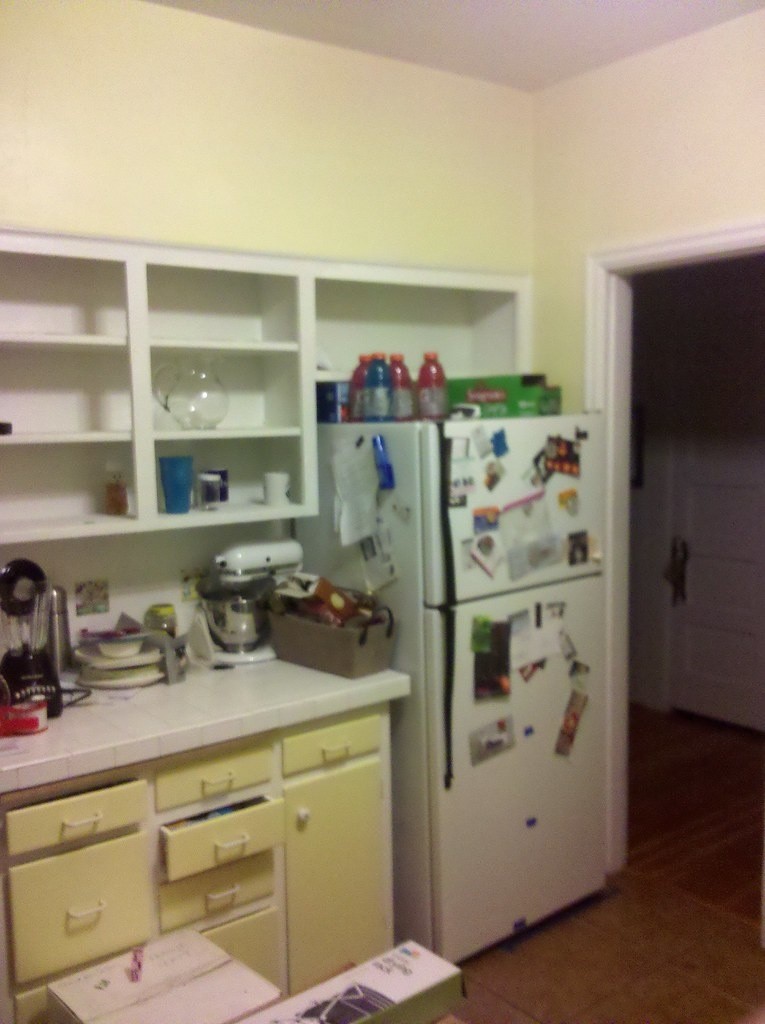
[74,646,166,689]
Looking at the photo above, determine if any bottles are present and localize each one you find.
[348,351,449,422]
[150,604,176,638]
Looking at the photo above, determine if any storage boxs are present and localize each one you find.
[442,373,562,416]
[45,927,284,1024]
[226,937,470,1023]
[316,380,353,424]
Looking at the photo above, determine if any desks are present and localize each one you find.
[1,640,412,1024]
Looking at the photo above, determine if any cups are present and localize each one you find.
[159,455,229,513]
[154,351,229,429]
[263,471,291,505]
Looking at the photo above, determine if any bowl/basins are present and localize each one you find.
[0,700,49,734]
[201,586,269,654]
[98,635,148,658]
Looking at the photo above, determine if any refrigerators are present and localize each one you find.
[294,411,606,966]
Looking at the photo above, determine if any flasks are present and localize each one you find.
[45,586,72,673]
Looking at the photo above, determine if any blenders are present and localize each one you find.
[207,539,304,667]
[1,559,63,718]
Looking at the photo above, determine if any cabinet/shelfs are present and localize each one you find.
[0,231,535,543]
[140,725,288,1006]
[280,702,385,1003]
[1,741,170,1024]
